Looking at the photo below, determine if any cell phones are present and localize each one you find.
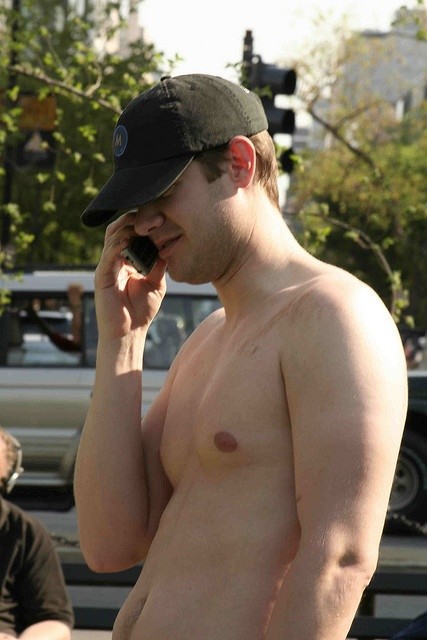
[117,219,160,277]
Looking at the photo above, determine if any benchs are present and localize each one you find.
[55,545,427,640]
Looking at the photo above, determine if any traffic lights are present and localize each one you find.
[248,61,297,173]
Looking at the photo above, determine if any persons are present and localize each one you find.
[69,70,412,640]
[0,427,77,640]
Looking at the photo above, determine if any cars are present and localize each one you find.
[382,370,426,533]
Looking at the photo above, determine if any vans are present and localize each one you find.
[2,268,225,492]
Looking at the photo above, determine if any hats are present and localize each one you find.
[80,73,269,229]
